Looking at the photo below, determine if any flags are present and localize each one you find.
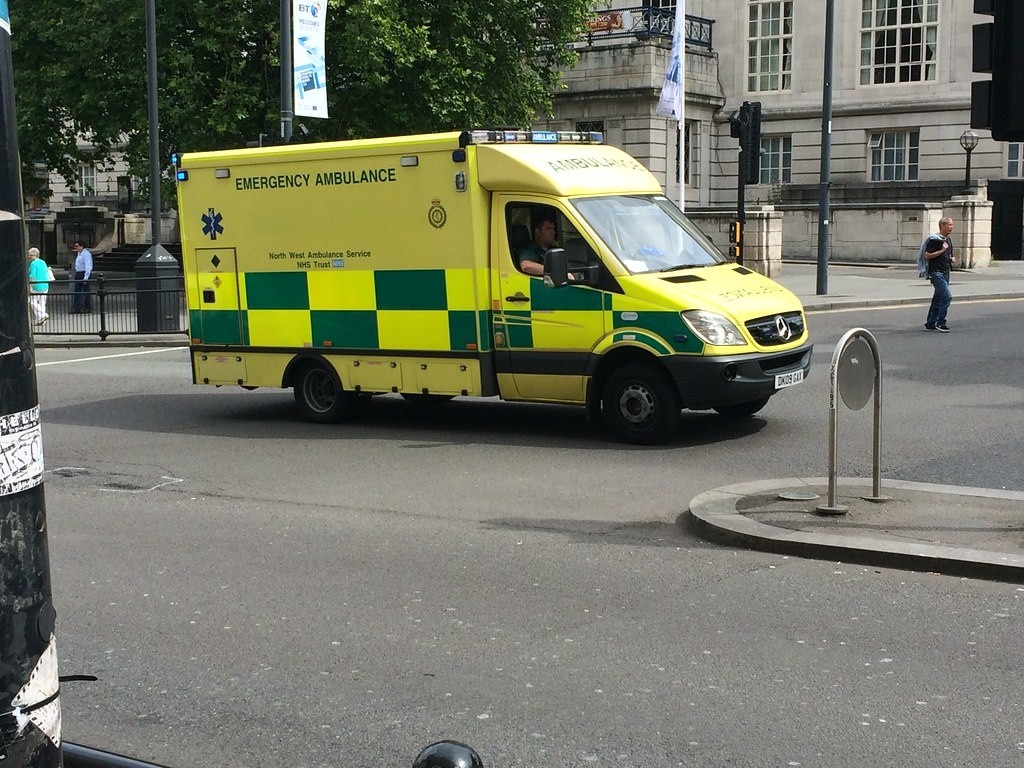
[293,0,328,118]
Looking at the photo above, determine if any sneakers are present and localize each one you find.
[925,323,939,331]
[935,324,951,331]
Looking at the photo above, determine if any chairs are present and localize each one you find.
[512,224,530,251]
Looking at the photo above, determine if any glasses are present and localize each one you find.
[75,245,80,248]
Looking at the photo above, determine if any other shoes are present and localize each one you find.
[69,309,80,314]
[80,309,91,313]
[34,318,45,326]
[39,313,50,325]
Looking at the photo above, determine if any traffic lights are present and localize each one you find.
[969,0,1024,143]
[728,221,740,244]
[729,247,740,257]
[983,176,1024,263]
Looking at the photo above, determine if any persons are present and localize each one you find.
[924,217,955,332]
[518,211,574,280]
[27,247,49,326]
[67,240,93,314]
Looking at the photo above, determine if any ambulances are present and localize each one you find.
[172,129,812,442]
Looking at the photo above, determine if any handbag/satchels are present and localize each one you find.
[47,266,55,281]
